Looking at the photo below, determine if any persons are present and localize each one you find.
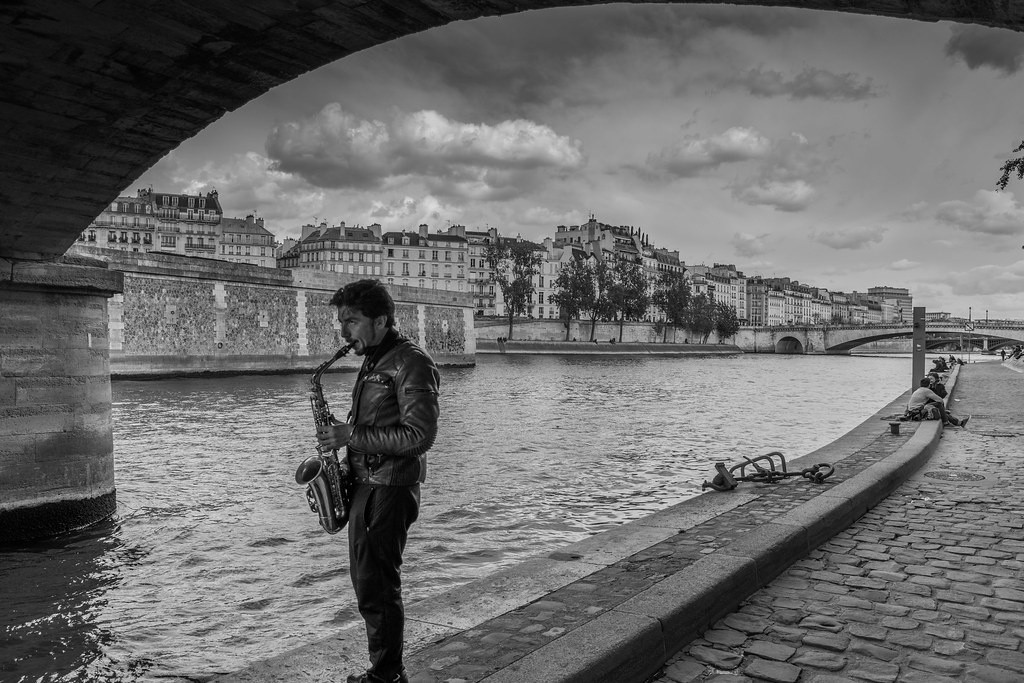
[1001,349,1005,362]
[908,373,972,429]
[949,354,956,362]
[310,277,440,683]
[929,356,948,372]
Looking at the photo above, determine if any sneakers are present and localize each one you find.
[347,662,410,683]
[960,415,971,428]
[943,423,956,428]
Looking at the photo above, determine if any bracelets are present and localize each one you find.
[348,425,358,445]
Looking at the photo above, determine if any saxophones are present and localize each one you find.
[294,344,351,535]
